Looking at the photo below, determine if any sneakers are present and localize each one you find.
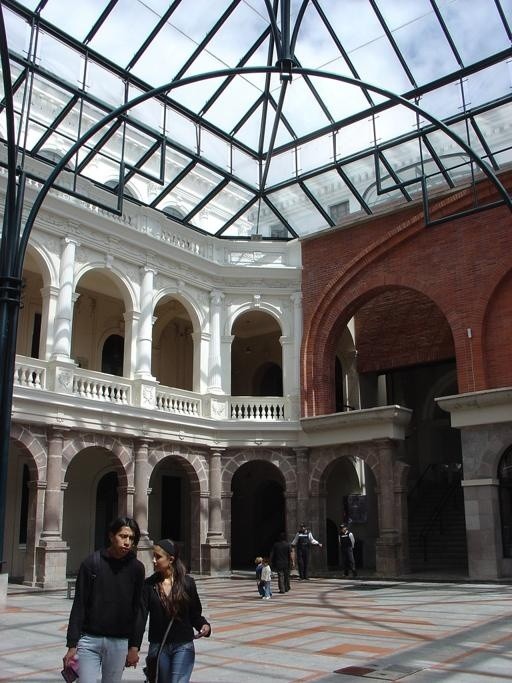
[344,573,357,576]
[299,576,309,580]
[279,586,290,594]
[262,593,272,599]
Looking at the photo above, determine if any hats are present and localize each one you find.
[156,538,176,554]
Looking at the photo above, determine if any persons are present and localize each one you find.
[125,539,211,682]
[260,557,274,600]
[62,516,146,682]
[254,555,265,596]
[338,522,357,577]
[270,532,293,593]
[291,521,322,581]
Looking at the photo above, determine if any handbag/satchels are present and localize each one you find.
[142,644,161,683]
